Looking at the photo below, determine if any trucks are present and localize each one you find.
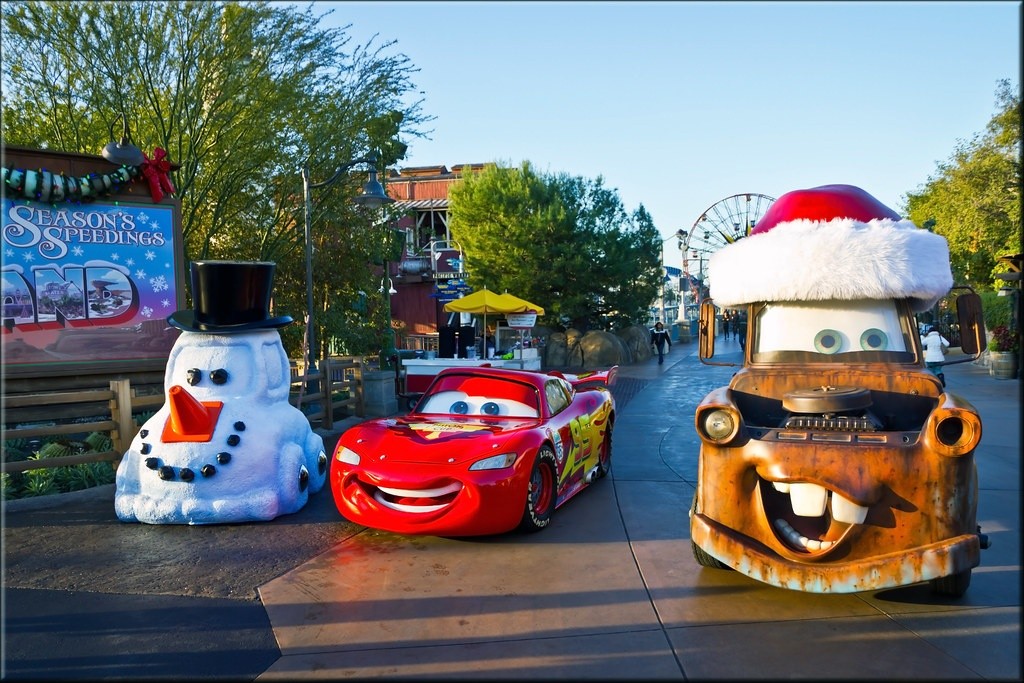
[685,180,989,597]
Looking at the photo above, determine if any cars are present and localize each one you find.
[110,256,329,526]
[331,364,617,541]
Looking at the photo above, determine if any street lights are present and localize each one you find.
[296,160,395,427]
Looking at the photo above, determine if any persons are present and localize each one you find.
[920,328,950,388]
[480,326,495,359]
[723,312,748,351]
[650,321,673,366]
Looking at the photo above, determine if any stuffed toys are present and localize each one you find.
[502,335,547,361]
